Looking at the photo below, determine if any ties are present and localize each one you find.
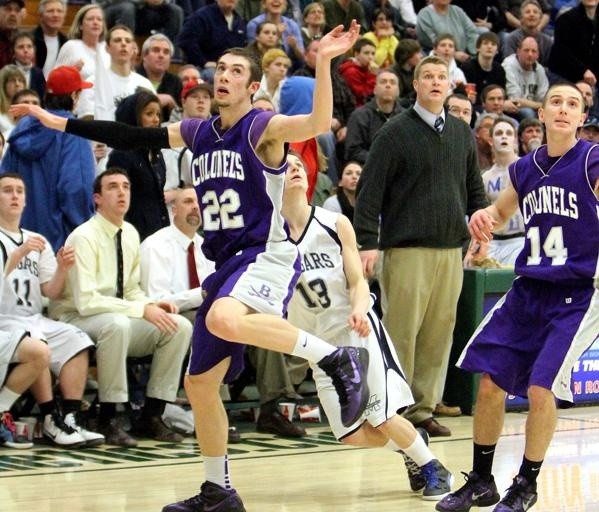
[114,229,125,302]
[187,241,202,292]
[434,115,446,133]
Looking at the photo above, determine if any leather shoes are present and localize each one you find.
[433,401,463,417]
[227,425,243,444]
[255,399,306,438]
[415,417,452,437]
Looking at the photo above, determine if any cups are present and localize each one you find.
[463,83,476,96]
[277,402,295,422]
[297,404,321,425]
[12,416,37,442]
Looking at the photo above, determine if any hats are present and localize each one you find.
[43,64,94,97]
[180,79,215,103]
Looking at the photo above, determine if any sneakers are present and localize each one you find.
[0,409,37,449]
[162,481,246,512]
[320,345,369,428]
[494,473,538,512]
[435,470,499,512]
[97,418,138,447]
[40,410,105,449]
[393,427,453,500]
[130,412,185,443]
